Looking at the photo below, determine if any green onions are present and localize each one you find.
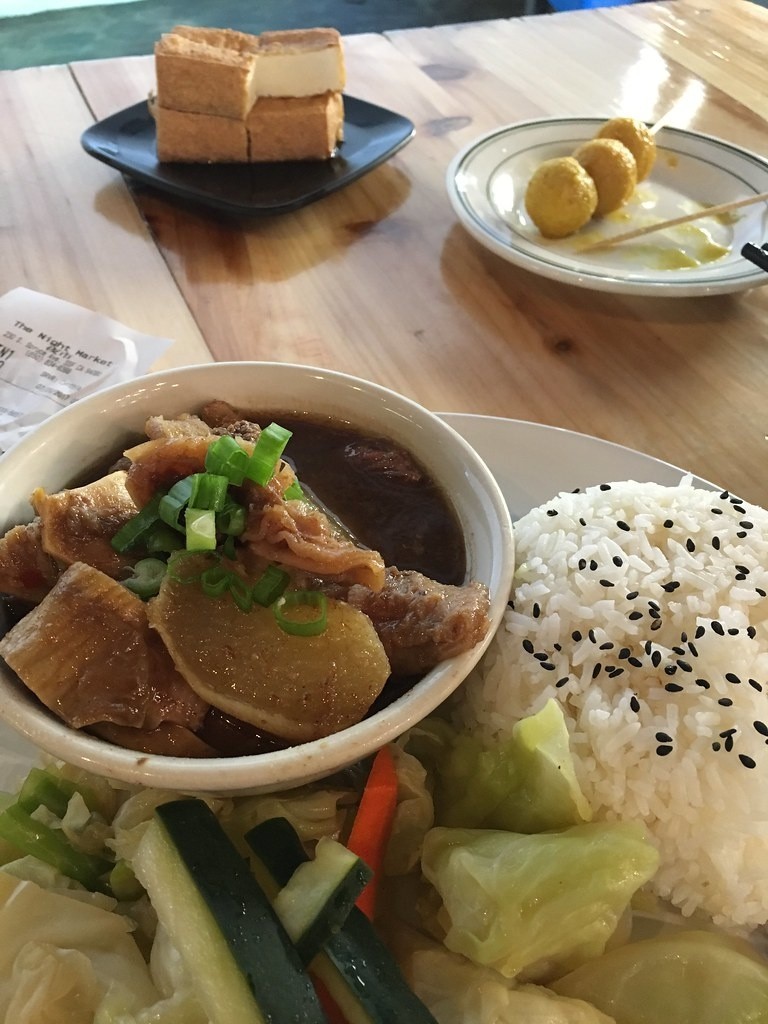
[107,422,329,637]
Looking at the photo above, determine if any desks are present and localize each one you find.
[0,0,768,512]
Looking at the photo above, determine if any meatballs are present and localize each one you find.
[525,118,656,240]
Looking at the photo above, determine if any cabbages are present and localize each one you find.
[0,698,768,1024]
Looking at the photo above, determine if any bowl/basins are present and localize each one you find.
[0,361,516,797]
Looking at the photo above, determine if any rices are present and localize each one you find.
[437,472,768,943]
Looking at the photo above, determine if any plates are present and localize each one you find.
[0,407,768,1024]
[447,116,768,297]
[80,92,415,216]
